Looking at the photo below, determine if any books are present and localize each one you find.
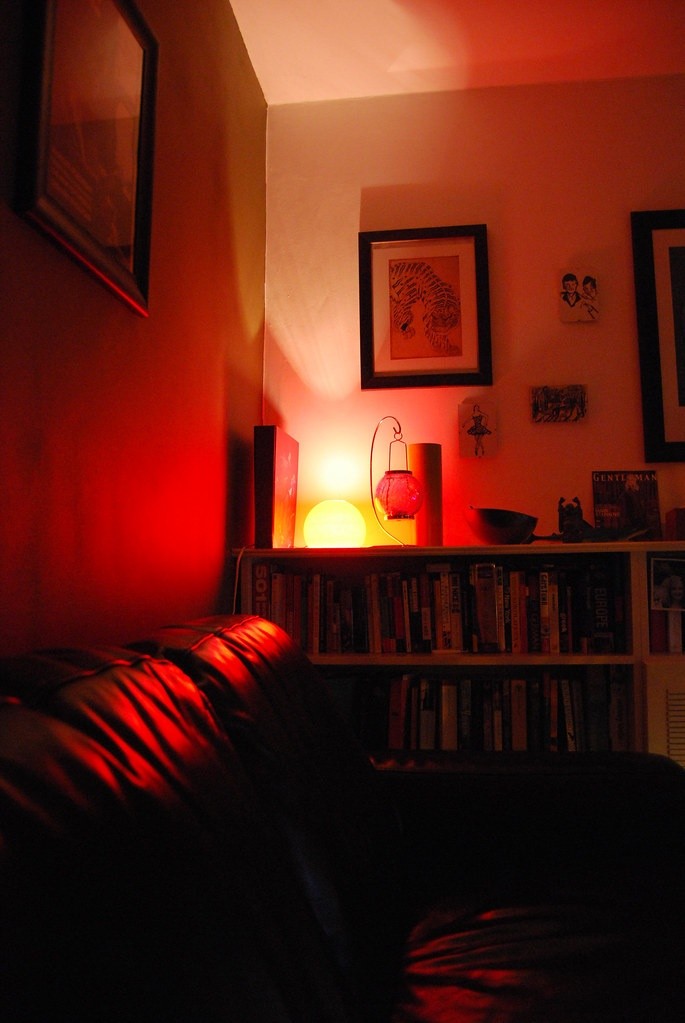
[592,470,663,542]
[252,559,629,752]
[648,554,682,654]
[254,425,299,548]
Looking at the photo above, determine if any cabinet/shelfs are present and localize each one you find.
[230,538,684,751]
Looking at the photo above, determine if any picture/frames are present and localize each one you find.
[629,209,685,464]
[15,0,159,317]
[357,223,493,389]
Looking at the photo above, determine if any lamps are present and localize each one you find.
[368,416,423,548]
[301,499,366,548]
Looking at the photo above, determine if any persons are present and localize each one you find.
[668,575,683,608]
[558,497,592,535]
[620,474,645,529]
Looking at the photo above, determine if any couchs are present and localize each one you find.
[0,613,685,1023]
